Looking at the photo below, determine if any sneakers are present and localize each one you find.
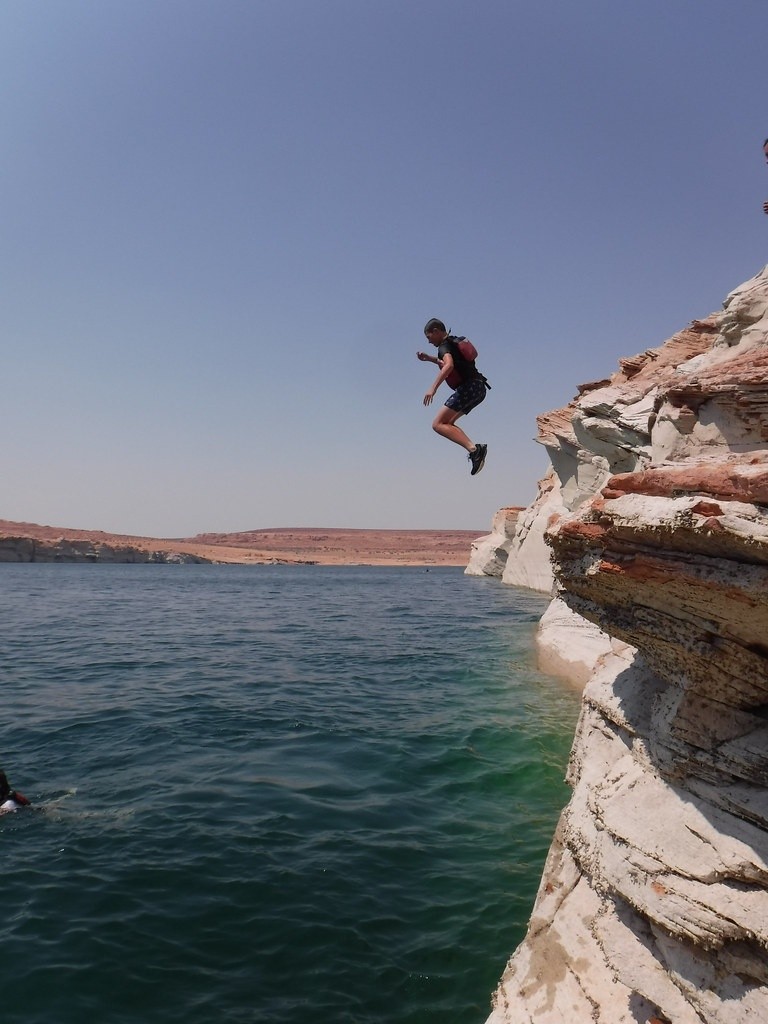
[468,442,487,475]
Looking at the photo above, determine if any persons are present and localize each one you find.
[416,318,491,475]
[0,774,22,815]
[763,139,768,215]
